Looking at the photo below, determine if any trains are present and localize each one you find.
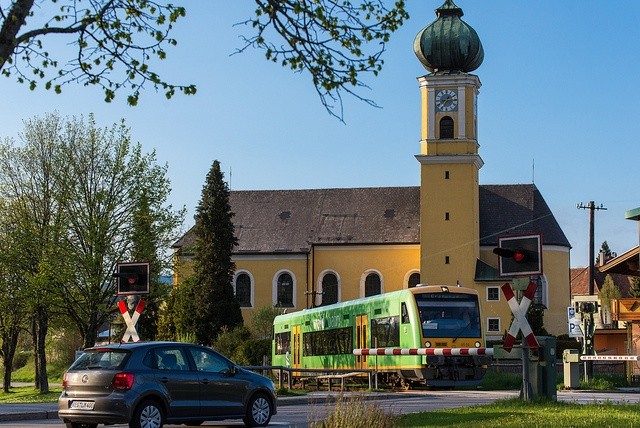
[270,283,490,390]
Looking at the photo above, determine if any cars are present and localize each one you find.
[57,341,278,428]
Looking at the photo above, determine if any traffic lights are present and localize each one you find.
[493,233,543,278]
[111,262,150,294]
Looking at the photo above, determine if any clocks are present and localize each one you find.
[435,88,458,111]
